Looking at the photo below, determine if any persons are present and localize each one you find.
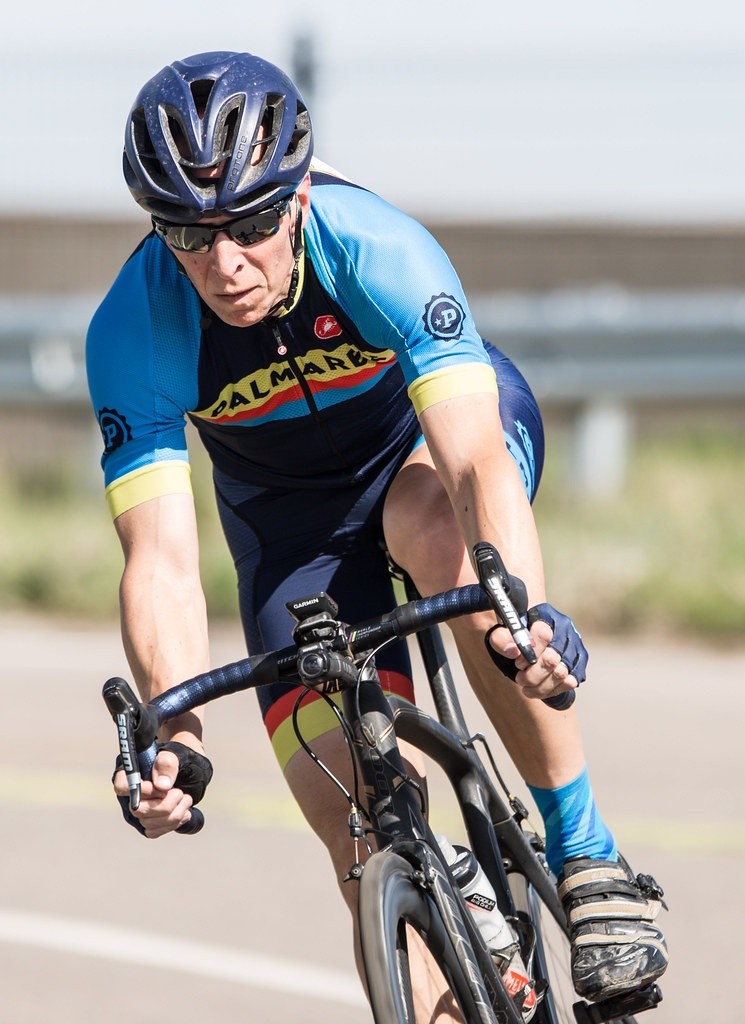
[84,50,668,1024]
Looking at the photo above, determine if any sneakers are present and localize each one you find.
[556,850,668,999]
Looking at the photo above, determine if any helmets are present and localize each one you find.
[123,51,314,224]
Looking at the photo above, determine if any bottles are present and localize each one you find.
[435,834,513,964]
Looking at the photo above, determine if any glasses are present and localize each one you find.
[151,192,297,253]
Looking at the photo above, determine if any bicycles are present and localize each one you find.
[101,519,662,1024]
